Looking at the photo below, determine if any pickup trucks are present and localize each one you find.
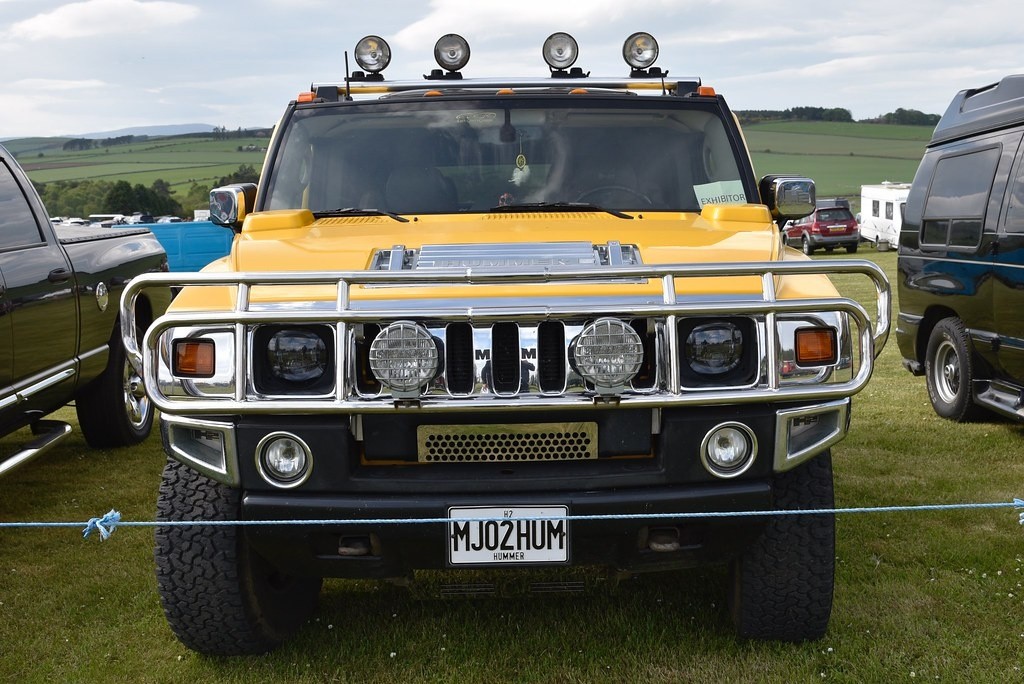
[0,141,179,492]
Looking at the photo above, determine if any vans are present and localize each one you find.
[855,183,915,253]
[893,73,1024,431]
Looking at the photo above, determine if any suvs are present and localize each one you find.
[122,30,891,662]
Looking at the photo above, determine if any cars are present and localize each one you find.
[52,208,214,227]
